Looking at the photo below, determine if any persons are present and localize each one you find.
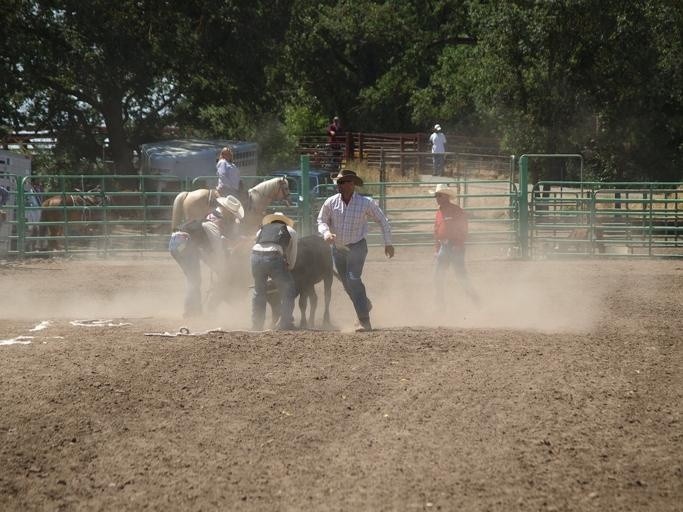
[428,184,483,317]
[429,124,447,177]
[318,169,394,332]
[326,116,345,162]
[169,147,297,331]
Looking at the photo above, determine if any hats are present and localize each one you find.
[262,211,293,227]
[428,183,457,201]
[331,168,363,186]
[433,123,442,130]
[216,194,245,220]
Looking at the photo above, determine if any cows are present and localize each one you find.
[559,229,605,255]
[247,232,350,332]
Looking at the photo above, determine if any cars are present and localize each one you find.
[260,167,333,222]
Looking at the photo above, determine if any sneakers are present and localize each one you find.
[355,298,374,331]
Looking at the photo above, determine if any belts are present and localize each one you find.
[251,249,282,257]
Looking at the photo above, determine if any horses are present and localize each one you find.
[171,173,293,240]
[38,187,140,256]
